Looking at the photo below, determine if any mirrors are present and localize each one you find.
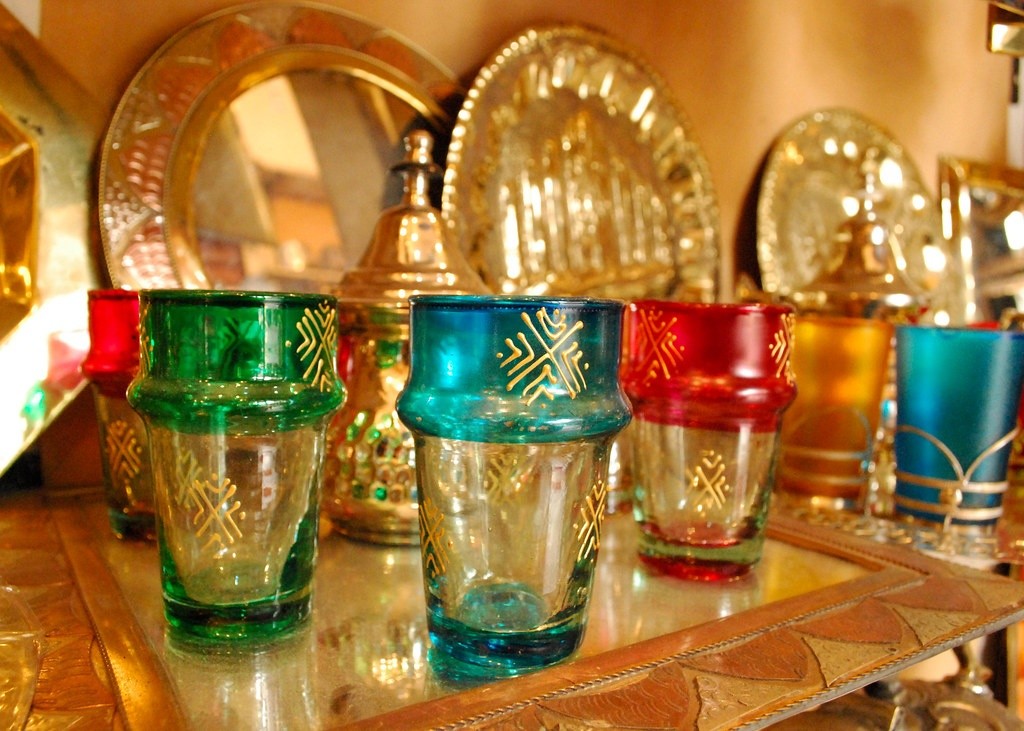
[940,156,1024,326]
[100,3,469,298]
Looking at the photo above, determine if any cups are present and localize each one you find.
[774,314,894,514]
[125,290,348,651]
[892,324,1024,526]
[617,297,796,584]
[82,290,158,544]
[394,296,632,668]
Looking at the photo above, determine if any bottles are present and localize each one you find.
[318,132,493,547]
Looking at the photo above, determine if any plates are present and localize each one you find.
[99,0,468,290]
[441,22,721,361]
[0,0,113,477]
[756,109,942,322]
